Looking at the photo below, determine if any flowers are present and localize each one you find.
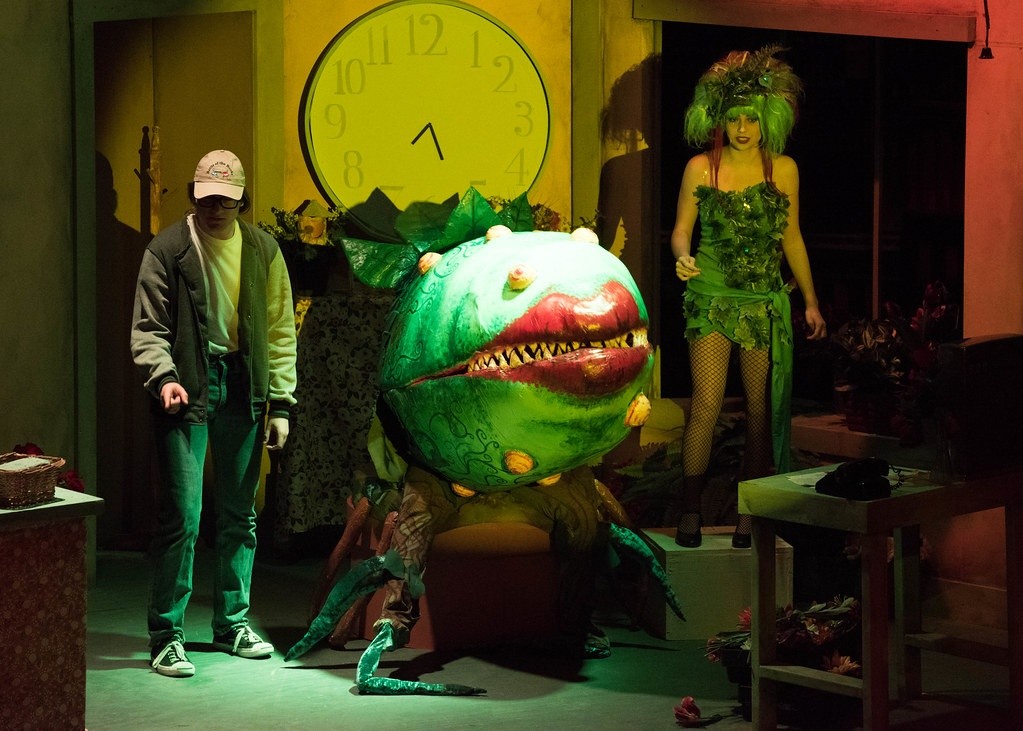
[700,593,864,673]
[794,282,959,417]
[672,696,739,729]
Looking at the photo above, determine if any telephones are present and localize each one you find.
[815,455,892,501]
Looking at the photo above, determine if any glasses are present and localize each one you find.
[197,195,240,209]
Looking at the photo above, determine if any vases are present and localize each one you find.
[843,397,947,442]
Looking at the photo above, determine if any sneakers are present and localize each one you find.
[213,625,274,658]
[151,639,196,676]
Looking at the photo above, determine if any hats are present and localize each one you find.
[194,150,246,200]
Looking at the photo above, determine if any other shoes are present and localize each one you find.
[676,528,702,547]
[732,531,750,547]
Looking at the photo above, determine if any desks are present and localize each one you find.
[738,460,1023,731]
[0,485,105,731]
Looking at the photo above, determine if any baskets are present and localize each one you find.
[0,452,66,506]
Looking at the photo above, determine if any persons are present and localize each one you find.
[130,149,298,677]
[671,80,828,549]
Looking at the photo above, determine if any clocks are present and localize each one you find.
[296,1,551,245]
